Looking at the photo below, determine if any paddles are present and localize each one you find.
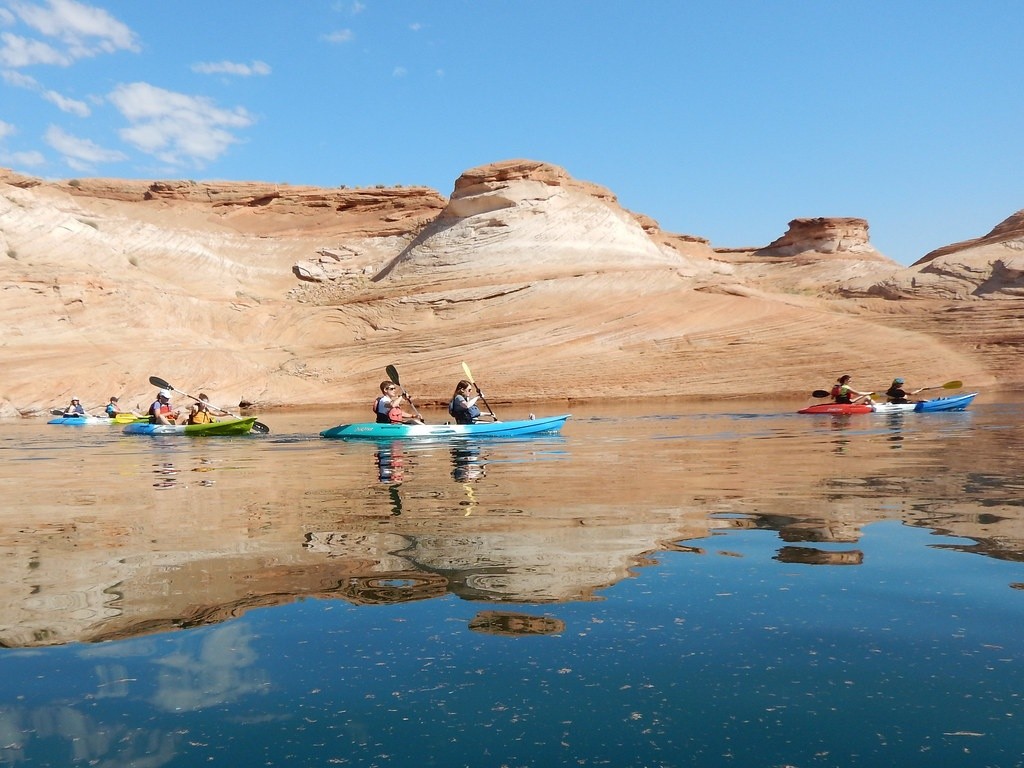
[811,390,874,398]
[922,380,963,390]
[148,375,269,434]
[460,360,498,423]
[52,410,81,416]
[386,364,427,422]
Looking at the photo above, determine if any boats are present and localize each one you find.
[122,416,263,438]
[794,391,981,416]
[319,411,572,438]
[46,414,153,426]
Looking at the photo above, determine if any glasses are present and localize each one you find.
[387,388,396,390]
[465,388,471,393]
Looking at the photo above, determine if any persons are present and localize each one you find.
[63,397,87,419]
[376,381,426,425]
[830,375,877,405]
[104,395,122,421]
[451,379,502,425]
[189,393,231,424]
[148,388,181,426]
[885,377,947,404]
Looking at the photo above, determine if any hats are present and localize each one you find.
[71,396,79,401]
[159,389,172,398]
[111,397,118,402]
[892,378,903,384]
[199,393,207,400]
